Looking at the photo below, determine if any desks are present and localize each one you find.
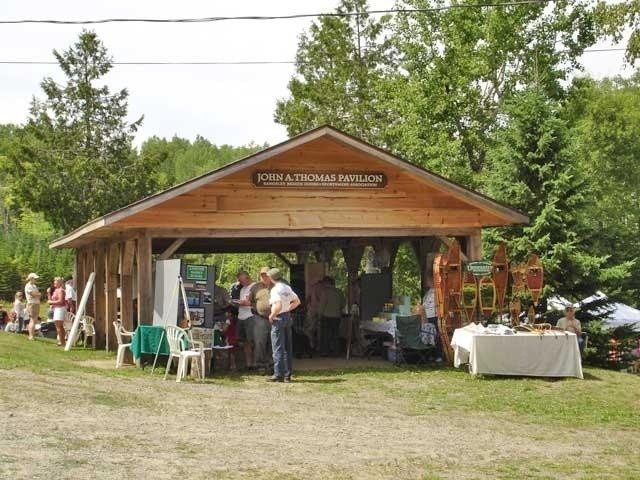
[447,325,586,381]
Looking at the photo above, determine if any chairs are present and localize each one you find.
[62,313,210,383]
[393,314,442,366]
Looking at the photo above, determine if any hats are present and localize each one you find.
[28,272,40,279]
[260,266,281,280]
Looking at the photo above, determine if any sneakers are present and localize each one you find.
[265,376,290,382]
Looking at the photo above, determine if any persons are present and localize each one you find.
[0,272,79,348]
[556,306,584,356]
[214,264,347,384]
[423,272,443,363]
